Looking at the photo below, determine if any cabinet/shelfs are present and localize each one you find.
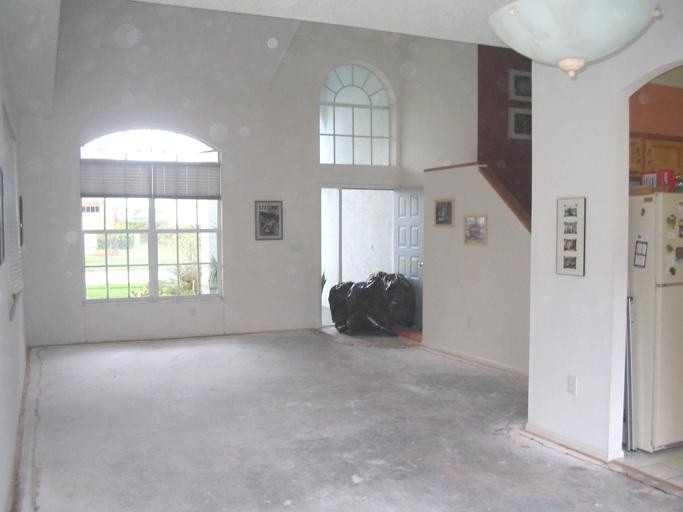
[629,137,683,179]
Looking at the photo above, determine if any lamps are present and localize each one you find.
[489,0,661,78]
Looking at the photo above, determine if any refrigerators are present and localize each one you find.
[623,186,682,452]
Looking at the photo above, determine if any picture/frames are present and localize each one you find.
[464,214,487,245]
[507,108,532,140]
[434,199,455,229]
[255,200,282,240]
[508,69,531,102]
[557,197,586,276]
